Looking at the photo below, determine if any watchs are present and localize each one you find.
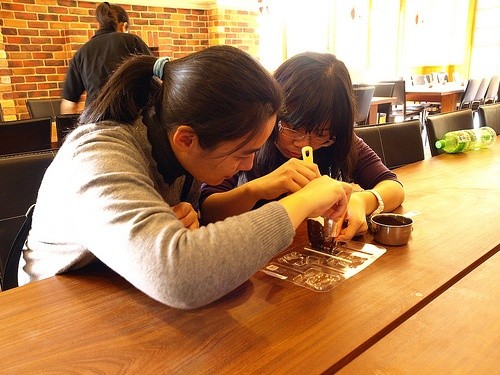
[366,190,384,219]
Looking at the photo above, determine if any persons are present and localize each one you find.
[198,52,405,243]
[17,45,353,312]
[61,2,163,111]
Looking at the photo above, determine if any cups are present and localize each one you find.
[304,218,334,252]
[369,213,413,245]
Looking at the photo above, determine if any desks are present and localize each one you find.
[0,134,500,375]
[368,96,397,125]
[405,88,465,114]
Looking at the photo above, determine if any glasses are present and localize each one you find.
[278,120,336,147]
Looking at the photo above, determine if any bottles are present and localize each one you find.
[434,126,498,155]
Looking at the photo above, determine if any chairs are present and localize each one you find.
[0,72,500,292]
